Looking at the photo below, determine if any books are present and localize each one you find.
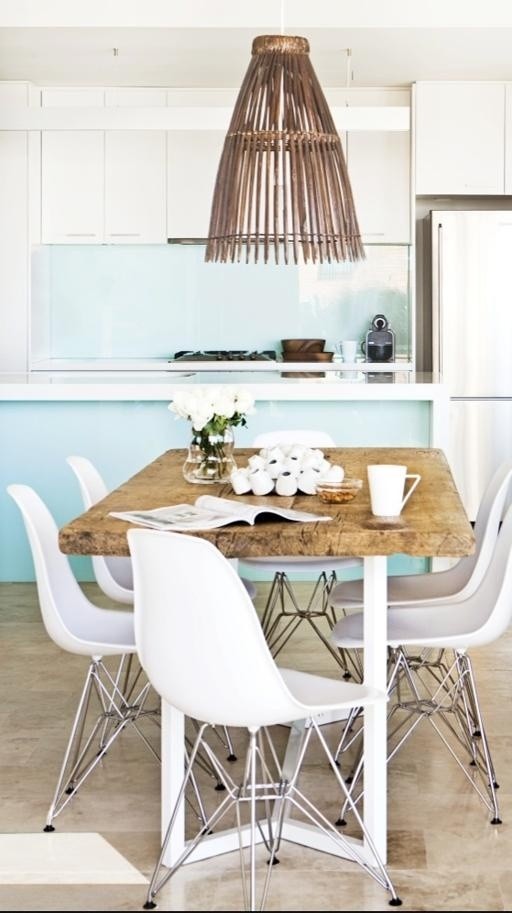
[108,493,331,532]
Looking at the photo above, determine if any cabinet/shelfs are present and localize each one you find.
[409,79,512,196]
[28,88,413,245]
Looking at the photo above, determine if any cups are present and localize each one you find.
[366,463,421,518]
[336,338,358,364]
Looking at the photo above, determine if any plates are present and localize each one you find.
[280,350,334,362]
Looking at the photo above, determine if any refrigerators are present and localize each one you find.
[420,207,511,523]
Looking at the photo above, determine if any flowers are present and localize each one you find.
[167,386,256,479]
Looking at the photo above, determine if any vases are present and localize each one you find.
[183,427,237,484]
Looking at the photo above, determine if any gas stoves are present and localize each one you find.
[168,348,277,363]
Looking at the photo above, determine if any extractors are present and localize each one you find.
[166,238,286,246]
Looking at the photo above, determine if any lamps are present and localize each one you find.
[206,0,367,265]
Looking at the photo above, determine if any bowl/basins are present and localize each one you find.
[281,338,327,352]
[312,478,364,502]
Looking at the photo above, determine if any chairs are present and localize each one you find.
[7,483,226,833]
[326,458,511,769]
[331,508,512,824]
[68,455,242,763]
[121,527,403,910]
[239,430,352,683]
